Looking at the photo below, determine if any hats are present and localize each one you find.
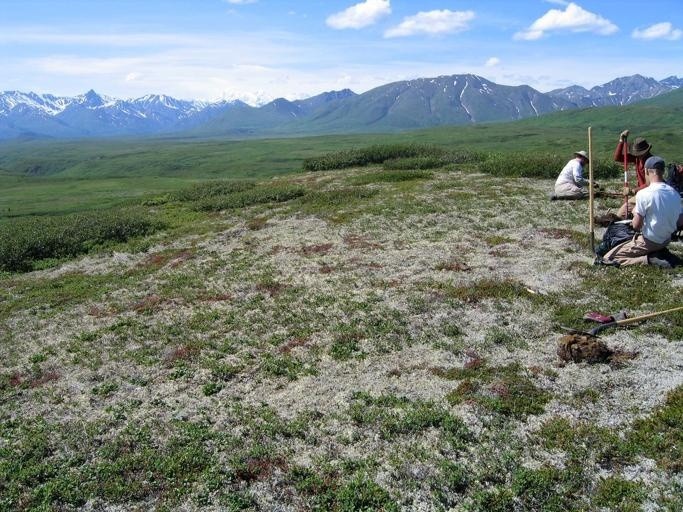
[627,136,652,157]
[642,155,665,170]
[572,150,590,164]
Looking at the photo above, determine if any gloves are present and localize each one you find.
[622,185,635,197]
[618,129,629,143]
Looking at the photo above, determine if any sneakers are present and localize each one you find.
[647,255,673,270]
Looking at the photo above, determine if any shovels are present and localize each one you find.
[587,127,598,265]
[561,307,682,336]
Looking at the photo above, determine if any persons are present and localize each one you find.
[550,149,600,201]
[613,129,656,221]
[602,155,683,270]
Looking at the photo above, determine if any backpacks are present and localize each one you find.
[595,221,638,256]
